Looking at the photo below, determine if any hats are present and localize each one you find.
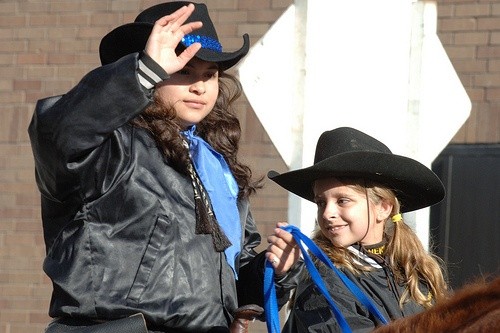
[267,127,447,212]
[99,1,249,74]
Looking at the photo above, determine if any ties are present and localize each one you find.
[179,124,242,280]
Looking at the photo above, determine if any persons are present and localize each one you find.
[26,1,312,333]
[267,125,455,333]
